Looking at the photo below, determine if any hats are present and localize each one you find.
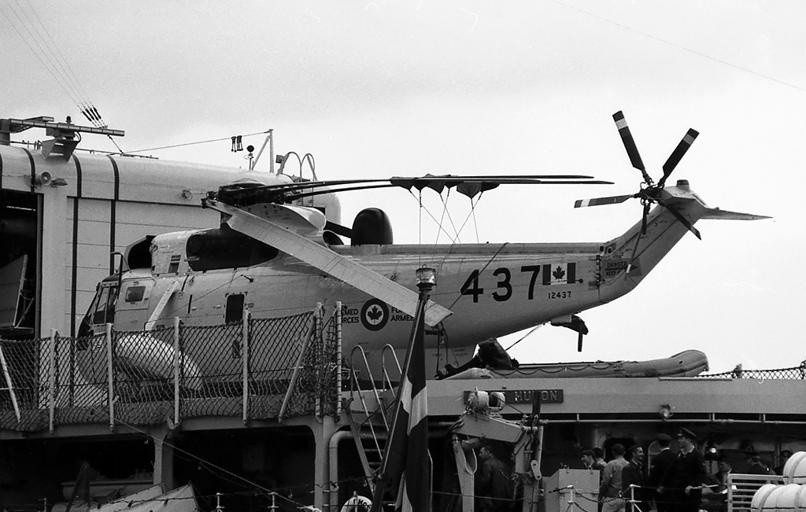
[656,433,673,444]
[676,427,697,439]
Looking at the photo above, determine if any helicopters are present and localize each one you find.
[76,111,774,397]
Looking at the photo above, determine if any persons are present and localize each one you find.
[475,445,513,512]
[580,428,793,512]
